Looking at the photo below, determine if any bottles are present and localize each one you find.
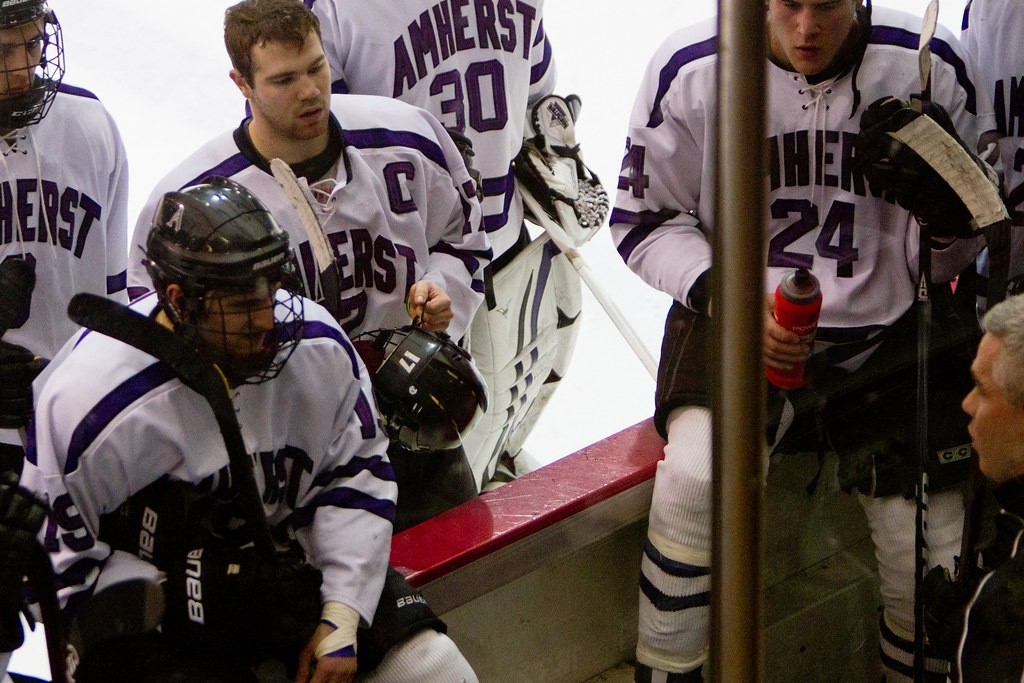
[763,268,823,391]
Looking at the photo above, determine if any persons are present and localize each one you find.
[0,0,1024,683]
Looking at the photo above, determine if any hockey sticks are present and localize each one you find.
[24,536,70,683]
[66,292,277,562]
[517,182,660,384]
[911,0,940,683]
[269,157,343,325]
[889,108,1015,683]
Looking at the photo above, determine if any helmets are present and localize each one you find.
[142,175,305,385]
[351,325,490,451]
[0,0,66,127]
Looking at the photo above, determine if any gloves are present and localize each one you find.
[164,542,322,663]
[856,94,1010,241]
[0,342,50,429]
